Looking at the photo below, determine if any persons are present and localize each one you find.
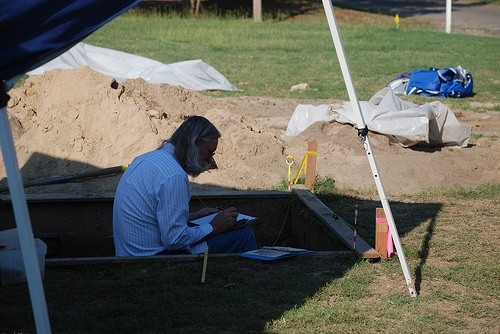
[111,116,261,256]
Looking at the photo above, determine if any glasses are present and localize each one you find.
[201,142,215,158]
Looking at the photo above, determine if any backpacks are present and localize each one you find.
[386,65,474,98]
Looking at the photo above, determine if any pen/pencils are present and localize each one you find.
[216,206,225,212]
